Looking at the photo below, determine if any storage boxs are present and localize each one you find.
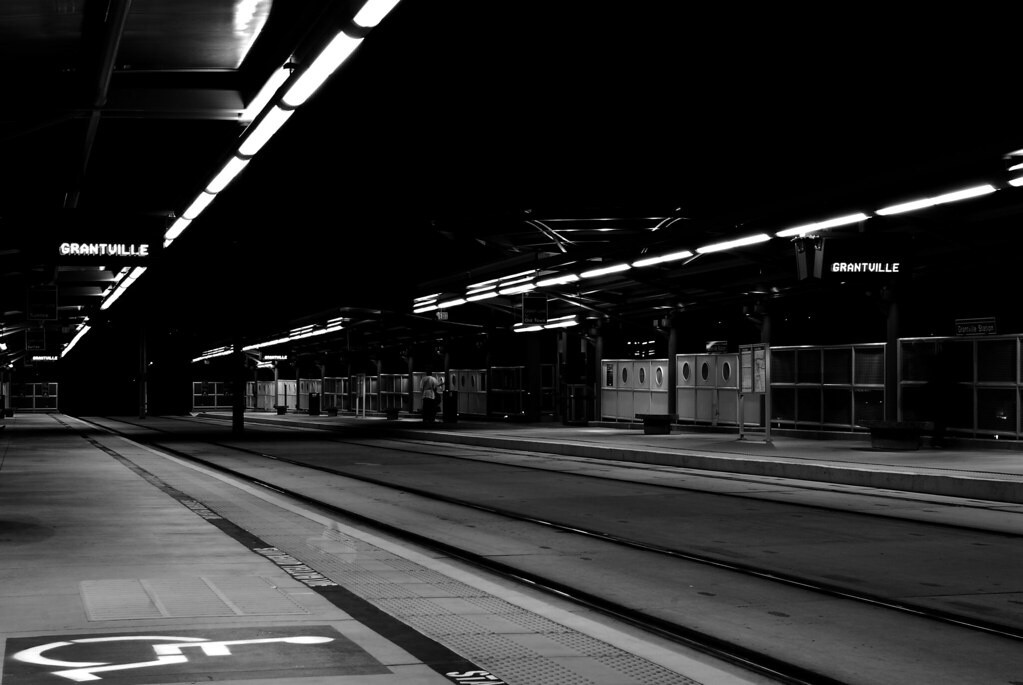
[870,426,923,450]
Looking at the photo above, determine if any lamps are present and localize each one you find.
[190,317,351,362]
[873,182,1001,216]
[646,276,683,310]
[534,273,581,288]
[60,325,91,357]
[578,259,634,279]
[465,268,541,290]
[480,326,488,334]
[499,274,536,296]
[165,0,402,251]
[744,279,782,315]
[695,232,774,255]
[436,309,449,320]
[774,211,873,238]
[434,340,446,354]
[585,311,601,320]
[631,249,693,268]
[1005,149,1023,187]
[438,298,466,309]
[99,266,147,310]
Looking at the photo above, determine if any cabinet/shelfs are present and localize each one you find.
[299,378,323,413]
[412,371,445,414]
[323,376,350,411]
[245,379,297,410]
[770,342,888,451]
[379,374,411,411]
[897,333,1023,443]
[9,382,58,410]
[351,375,380,414]
[490,366,525,421]
[193,381,234,410]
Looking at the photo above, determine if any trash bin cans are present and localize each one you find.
[443,390,459,430]
[309,392,321,417]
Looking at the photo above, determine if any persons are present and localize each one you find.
[420,371,444,426]
